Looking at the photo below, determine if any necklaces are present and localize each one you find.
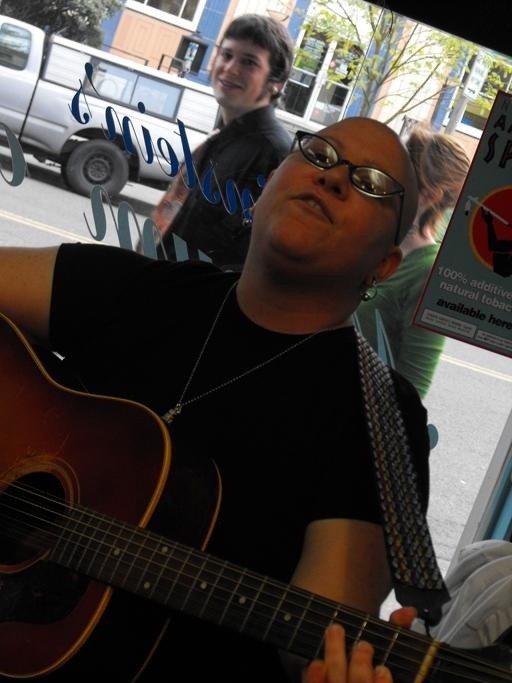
[161,281,335,424]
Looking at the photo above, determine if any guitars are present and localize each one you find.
[0,311,512,683]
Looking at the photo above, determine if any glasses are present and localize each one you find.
[288,129,406,248]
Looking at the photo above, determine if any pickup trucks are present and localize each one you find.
[1,16,215,199]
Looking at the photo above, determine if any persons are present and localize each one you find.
[135,12,296,272]
[0,116,432,679]
[350,125,472,400]
